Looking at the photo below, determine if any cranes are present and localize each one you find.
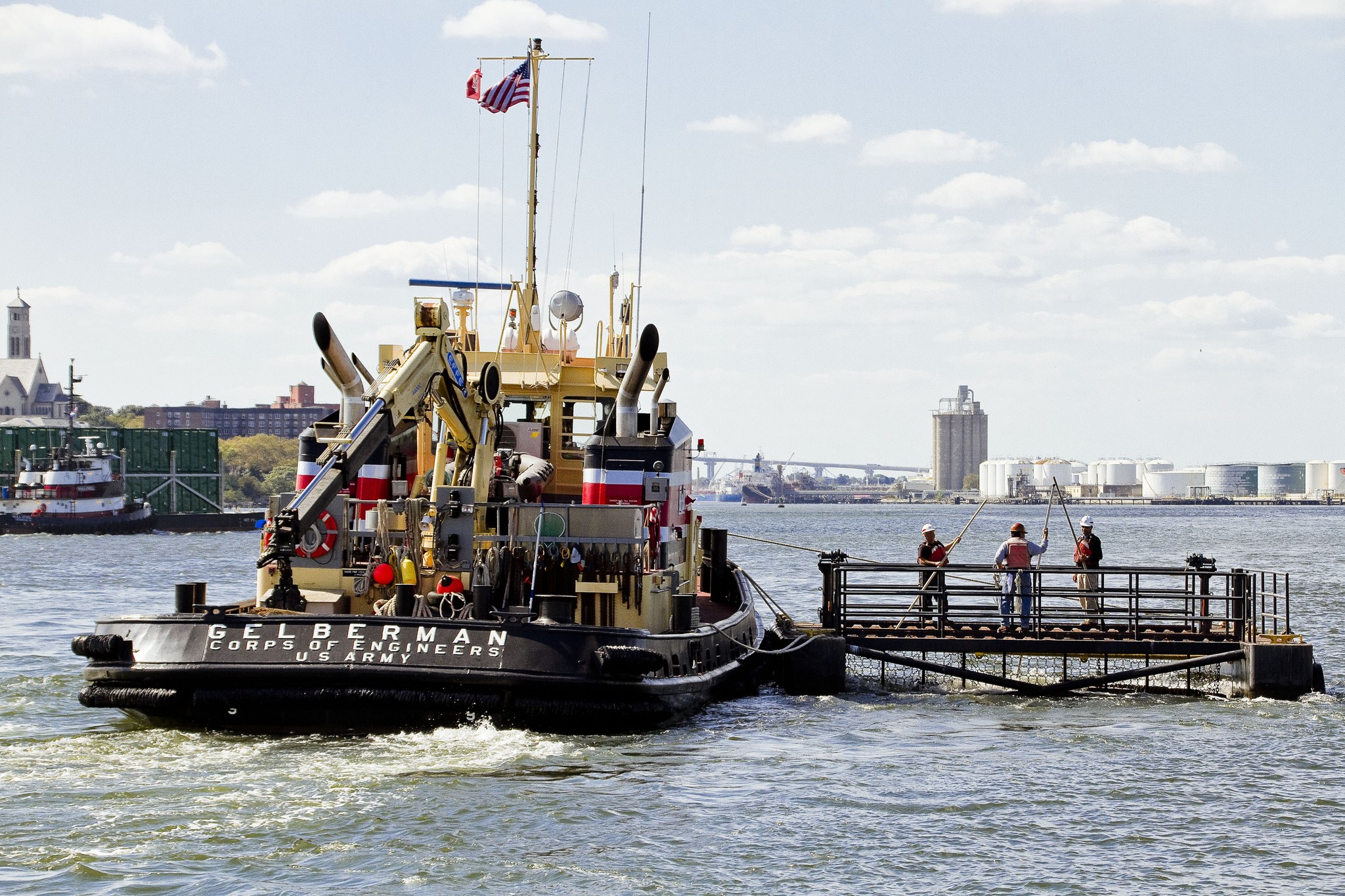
[707,462,726,490]
[781,452,794,477]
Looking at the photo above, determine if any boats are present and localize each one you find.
[768,498,775,504]
[741,453,779,503]
[0,358,157,536]
[740,497,747,506]
[777,505,785,508]
[71,37,763,740]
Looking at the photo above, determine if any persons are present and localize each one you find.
[917,524,962,623]
[1072,516,1103,626]
[994,523,1048,633]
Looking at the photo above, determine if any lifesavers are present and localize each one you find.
[295,510,338,558]
[428,589,473,618]
[649,507,661,555]
[112,473,119,481]
[264,517,274,551]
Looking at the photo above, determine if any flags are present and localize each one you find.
[466,68,481,101]
[478,51,530,114]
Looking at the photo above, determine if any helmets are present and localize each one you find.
[1010,523,1028,534]
[921,524,936,534]
[1080,515,1094,526]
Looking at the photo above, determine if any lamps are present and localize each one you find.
[672,527,683,542]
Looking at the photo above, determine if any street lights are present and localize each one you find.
[1131,489,1133,498]
[1087,489,1089,498]
[1109,489,1112,498]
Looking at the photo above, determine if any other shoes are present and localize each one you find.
[944,619,953,624]
[1000,626,1009,632]
[1079,621,1098,626]
[924,619,936,623]
[1019,628,1029,633]
